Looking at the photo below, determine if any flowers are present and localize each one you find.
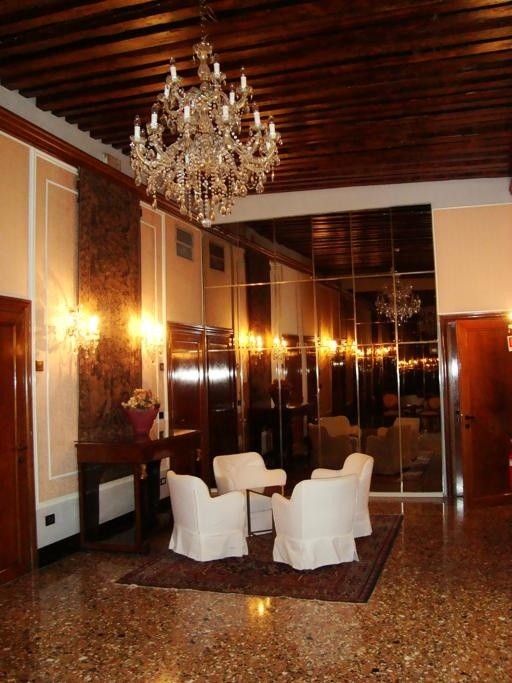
[268,378,293,389]
[124,387,160,408]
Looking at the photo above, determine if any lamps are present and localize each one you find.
[375,247,422,324]
[49,303,102,361]
[128,0,285,228]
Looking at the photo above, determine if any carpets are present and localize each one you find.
[113,511,404,601]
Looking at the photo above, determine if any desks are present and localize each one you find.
[246,399,314,470]
[73,423,204,554]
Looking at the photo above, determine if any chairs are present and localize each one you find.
[269,472,359,570]
[161,468,249,566]
[305,413,436,484]
[311,450,378,538]
[211,450,288,535]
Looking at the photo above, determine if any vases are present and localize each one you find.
[126,407,157,434]
[270,388,289,407]
